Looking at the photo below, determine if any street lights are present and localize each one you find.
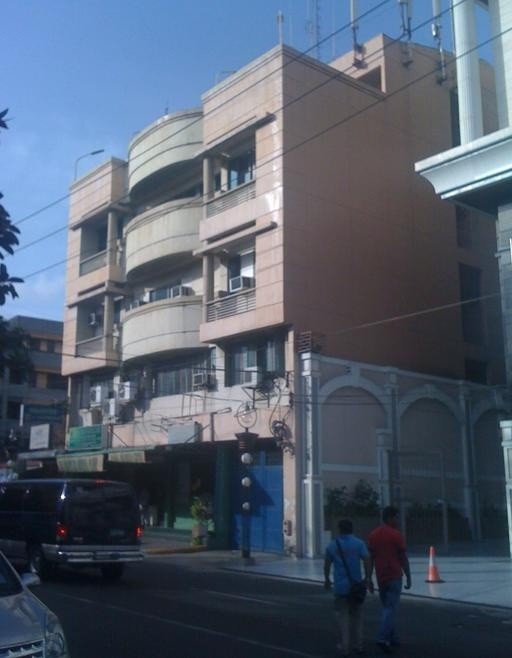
[227,401,263,557]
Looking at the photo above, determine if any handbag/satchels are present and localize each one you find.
[346,580,370,603]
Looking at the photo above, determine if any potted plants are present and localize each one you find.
[190,495,214,539]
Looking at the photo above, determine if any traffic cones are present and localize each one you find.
[425,545,445,583]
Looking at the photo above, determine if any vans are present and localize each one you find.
[0,477,144,582]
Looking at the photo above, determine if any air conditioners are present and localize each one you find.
[87,312,102,327]
[240,366,263,387]
[89,381,138,418]
[228,276,251,294]
[170,285,193,298]
[191,373,206,387]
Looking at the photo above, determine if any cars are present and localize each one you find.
[0,550,70,658]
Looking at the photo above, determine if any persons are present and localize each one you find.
[322,518,370,655]
[365,505,413,653]
